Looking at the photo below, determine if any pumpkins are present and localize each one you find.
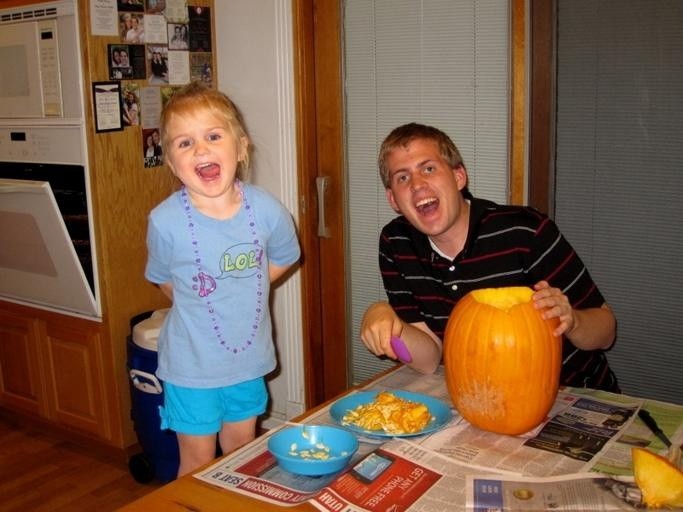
[442,287,564,436]
[632,448,683,509]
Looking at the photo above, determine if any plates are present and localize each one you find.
[332,389,450,438]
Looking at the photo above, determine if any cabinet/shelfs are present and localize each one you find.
[0,302,124,466]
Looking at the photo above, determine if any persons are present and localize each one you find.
[143,83,302,479]
[149,49,168,82]
[112,46,134,79]
[171,25,188,48]
[147,132,162,159]
[201,59,212,81]
[120,87,140,127]
[122,14,147,43]
[359,123,620,393]
[147,0,165,13]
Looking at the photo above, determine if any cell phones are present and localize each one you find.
[350,451,394,486]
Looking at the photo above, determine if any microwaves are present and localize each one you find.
[0,0,80,123]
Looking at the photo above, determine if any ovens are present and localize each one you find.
[1,124,102,322]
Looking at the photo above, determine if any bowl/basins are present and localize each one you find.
[268,425,358,477]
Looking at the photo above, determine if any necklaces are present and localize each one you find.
[183,180,263,353]
